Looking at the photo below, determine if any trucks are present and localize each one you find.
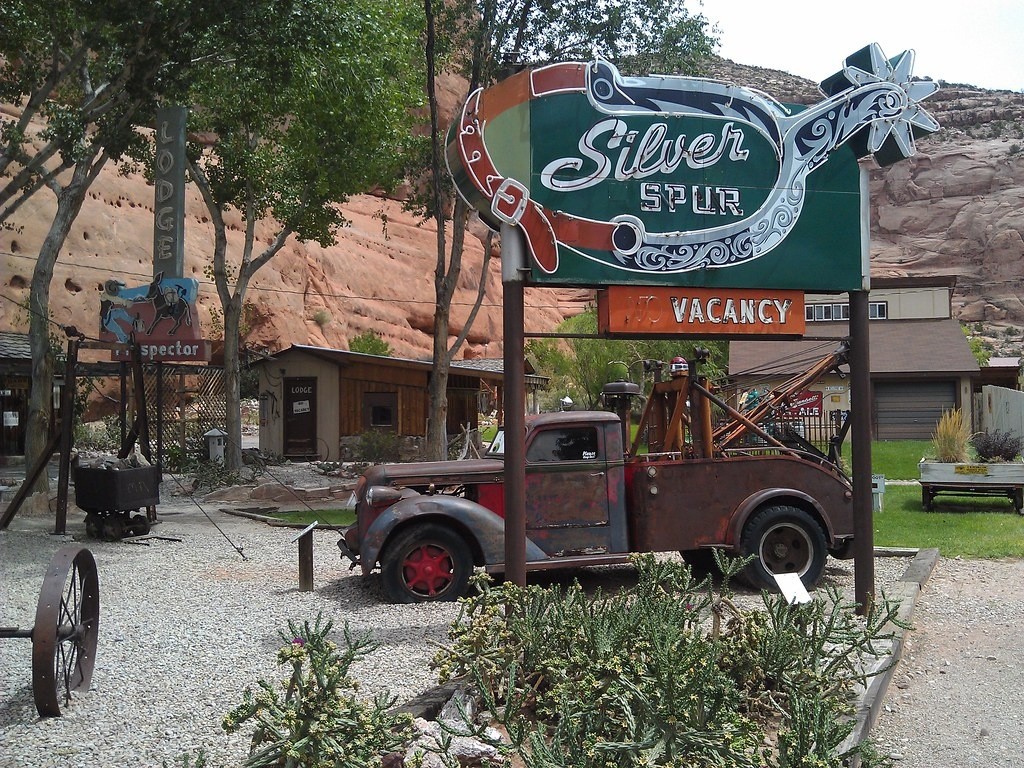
[337,357,855,605]
